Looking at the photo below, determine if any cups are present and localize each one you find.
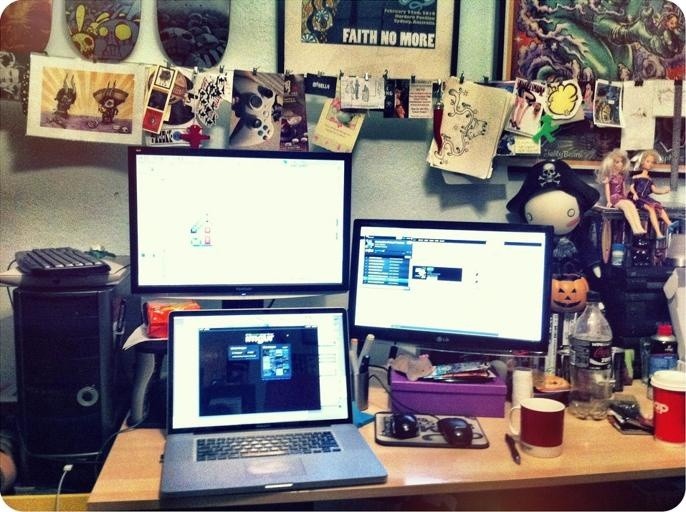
[648,368,685,448]
[349,374,368,414]
[507,398,565,459]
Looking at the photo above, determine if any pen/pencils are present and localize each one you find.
[349,334,375,374]
[609,403,654,428]
[443,379,465,383]
[504,433,521,465]
[112,297,127,381]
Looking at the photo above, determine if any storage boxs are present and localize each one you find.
[386,365,506,420]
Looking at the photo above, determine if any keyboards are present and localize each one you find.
[13,245,109,275]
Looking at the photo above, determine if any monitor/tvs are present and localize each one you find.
[127,145,352,296]
[339,218,554,355]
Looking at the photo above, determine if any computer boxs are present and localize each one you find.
[14,254,139,500]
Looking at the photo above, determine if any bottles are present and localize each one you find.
[632,238,649,267]
[609,243,625,267]
[567,291,612,419]
[648,324,679,400]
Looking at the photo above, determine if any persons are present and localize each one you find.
[605,152,647,235]
[630,148,674,240]
[511,88,540,128]
[506,160,603,283]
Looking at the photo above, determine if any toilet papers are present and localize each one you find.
[511,367,534,410]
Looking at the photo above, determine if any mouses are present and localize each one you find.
[438,413,474,449]
[392,408,416,438]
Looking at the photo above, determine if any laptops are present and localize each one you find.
[158,312,386,502]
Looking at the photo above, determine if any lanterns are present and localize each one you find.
[547,276,588,312]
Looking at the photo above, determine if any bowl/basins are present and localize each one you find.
[286,115,305,126]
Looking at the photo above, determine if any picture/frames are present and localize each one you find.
[273,0,460,84]
[491,2,685,176]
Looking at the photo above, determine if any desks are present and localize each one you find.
[84,374,686,512]
